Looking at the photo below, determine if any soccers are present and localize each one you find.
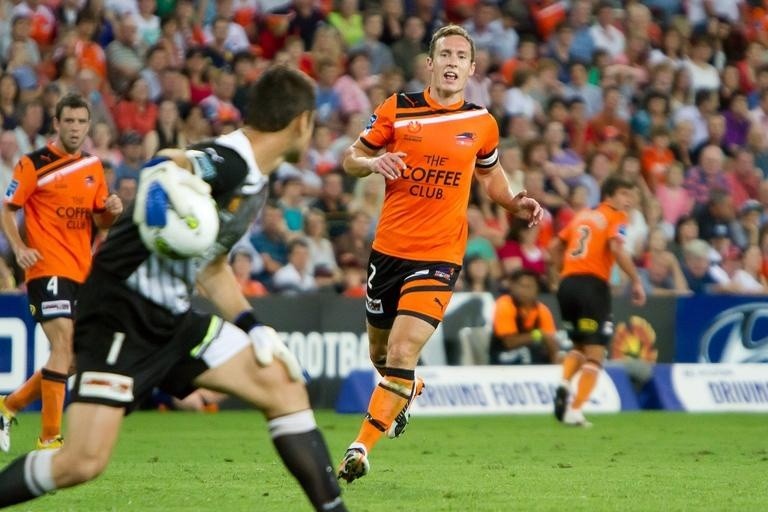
[139,174,220,258]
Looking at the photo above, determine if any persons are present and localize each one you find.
[546,177,649,430]
[333,22,545,484]
[488,268,561,364]
[1,0,768,294]
[0,63,357,511]
[0,90,127,454]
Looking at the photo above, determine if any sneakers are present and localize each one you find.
[336,443,370,484]
[36,433,65,450]
[0,394,14,452]
[385,377,425,439]
[554,380,593,427]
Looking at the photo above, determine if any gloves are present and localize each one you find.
[132,155,212,229]
[234,309,302,382]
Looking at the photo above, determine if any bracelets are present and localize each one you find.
[531,329,542,342]
[231,308,263,331]
